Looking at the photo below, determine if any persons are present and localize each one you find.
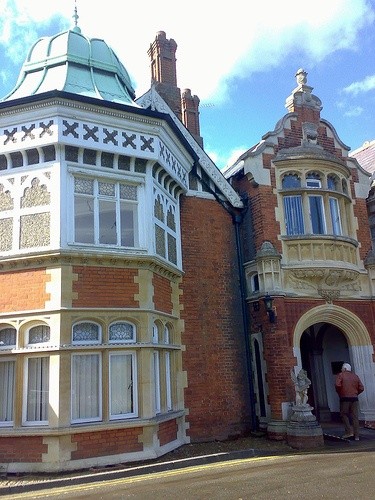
[335,363,364,440]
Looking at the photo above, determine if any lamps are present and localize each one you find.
[261,291,278,326]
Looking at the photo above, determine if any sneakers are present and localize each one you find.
[343,433,354,438]
[354,435,359,440]
